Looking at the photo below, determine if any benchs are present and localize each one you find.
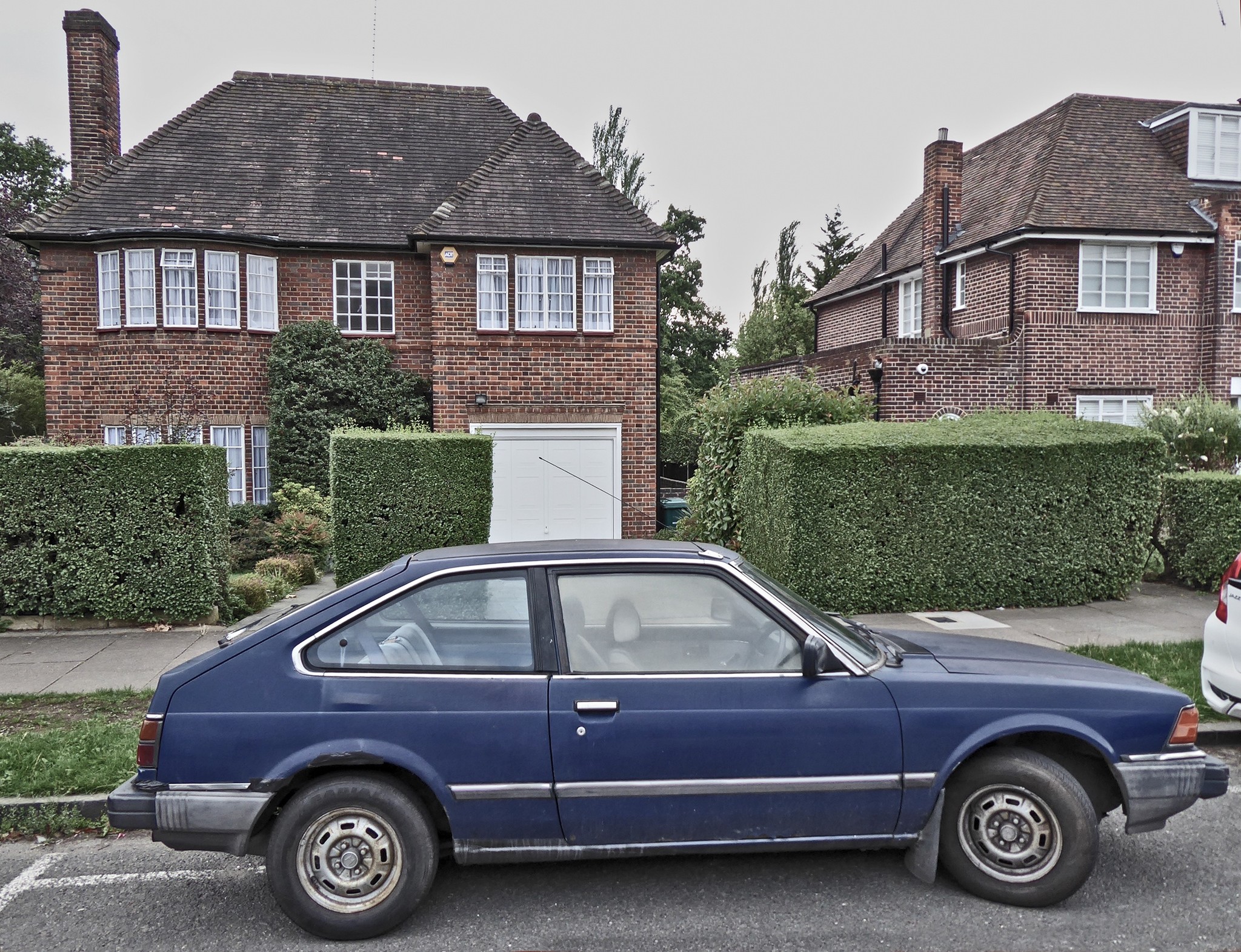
[357,623,443,665]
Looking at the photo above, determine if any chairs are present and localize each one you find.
[605,599,658,671]
[563,593,611,672]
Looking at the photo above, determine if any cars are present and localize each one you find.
[1200,547,1241,723]
[106,539,1230,942]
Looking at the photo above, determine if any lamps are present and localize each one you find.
[475,394,487,405]
